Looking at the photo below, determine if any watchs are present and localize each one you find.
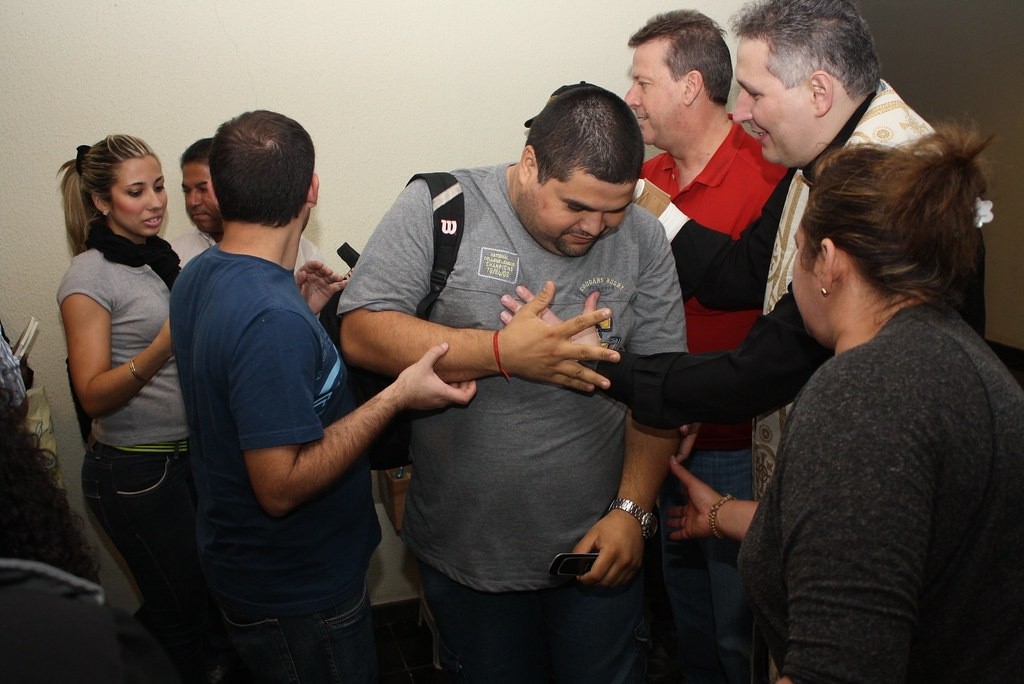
[607,498,658,540]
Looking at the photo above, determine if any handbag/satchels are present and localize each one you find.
[317,172,466,470]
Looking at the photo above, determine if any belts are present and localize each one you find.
[114,438,193,452]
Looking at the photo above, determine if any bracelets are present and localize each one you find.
[494,328,512,381]
[128,356,148,383]
[707,493,737,539]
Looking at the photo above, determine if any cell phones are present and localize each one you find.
[548,552,600,579]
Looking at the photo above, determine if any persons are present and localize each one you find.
[0,318,187,684]
[665,121,1024,684]
[55,135,221,683]
[170,110,477,684]
[337,85,689,683]
[501,0,988,684]
[625,9,788,684]
[171,138,338,279]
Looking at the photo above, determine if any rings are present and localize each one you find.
[681,517,686,526]
